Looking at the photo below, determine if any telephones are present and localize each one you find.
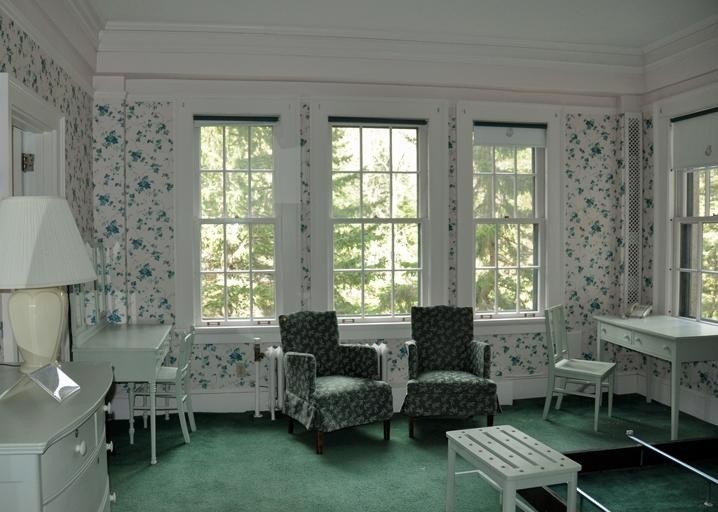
[625,303,653,318]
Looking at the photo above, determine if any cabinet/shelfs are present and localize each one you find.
[0,362,119,512]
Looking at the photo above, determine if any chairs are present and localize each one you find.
[539,301,618,436]
[125,330,200,446]
[402,303,497,439]
[274,308,393,455]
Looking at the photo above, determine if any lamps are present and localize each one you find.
[0,190,99,379]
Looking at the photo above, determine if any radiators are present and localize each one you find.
[247,340,389,424]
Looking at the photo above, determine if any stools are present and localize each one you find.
[436,420,584,512]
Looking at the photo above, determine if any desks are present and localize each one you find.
[73,319,176,467]
[586,306,717,443]
